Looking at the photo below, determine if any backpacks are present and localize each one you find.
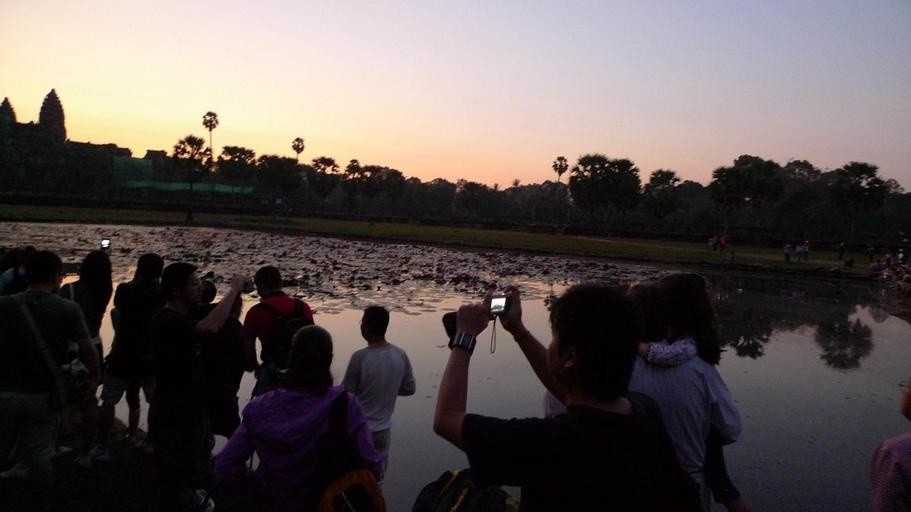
[258,298,309,368]
[299,392,386,512]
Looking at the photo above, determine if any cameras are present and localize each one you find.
[490,292,507,315]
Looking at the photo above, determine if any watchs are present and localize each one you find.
[451,332,477,355]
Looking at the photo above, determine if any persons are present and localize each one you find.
[869,374,911,512]
[781,239,813,266]
[340,305,415,489]
[214,324,382,512]
[148,263,247,512]
[433,283,704,512]
[238,266,315,399]
[90,254,165,462]
[211,296,244,436]
[1,251,101,479]
[192,280,216,322]
[57,249,113,466]
[637,274,750,512]
[0,246,37,296]
[543,273,742,511]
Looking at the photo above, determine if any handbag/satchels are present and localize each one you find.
[43,358,92,404]
